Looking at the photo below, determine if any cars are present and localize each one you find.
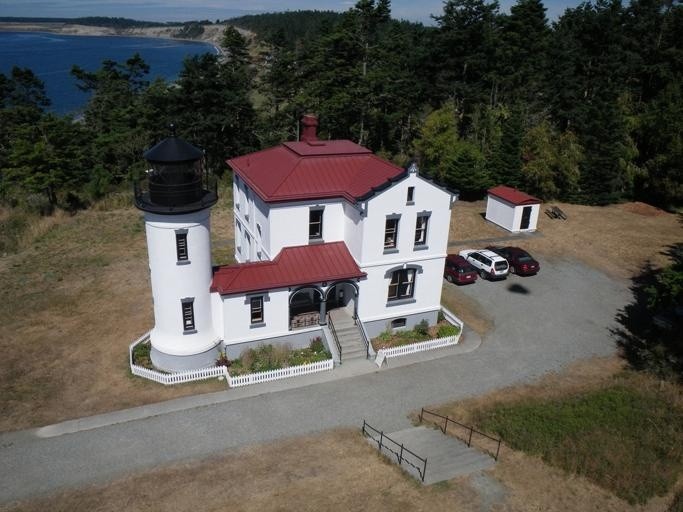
[444,253,477,286]
[484,245,540,276]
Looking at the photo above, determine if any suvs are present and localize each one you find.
[458,249,511,280]
